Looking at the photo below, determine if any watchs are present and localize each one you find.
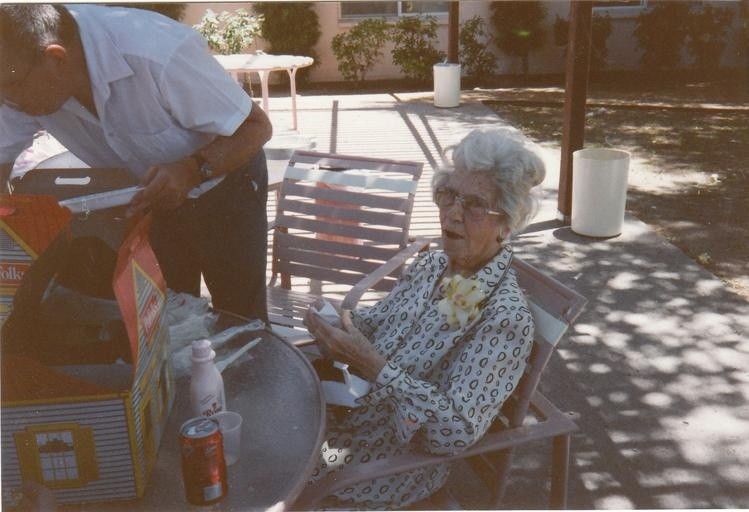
[193,152,214,183]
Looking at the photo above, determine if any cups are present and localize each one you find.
[209,410,246,468]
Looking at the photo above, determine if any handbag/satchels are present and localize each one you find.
[12,215,131,364]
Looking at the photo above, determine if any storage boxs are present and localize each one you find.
[0,166,142,328]
[0,232,177,505]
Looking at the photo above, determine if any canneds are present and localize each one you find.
[178,416,228,506]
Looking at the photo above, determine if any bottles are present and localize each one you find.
[189,338,227,417]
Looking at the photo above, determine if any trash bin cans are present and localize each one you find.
[570,149,631,239]
[432,63,463,109]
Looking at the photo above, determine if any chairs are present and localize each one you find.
[295,256,587,511]
[266,148,425,345]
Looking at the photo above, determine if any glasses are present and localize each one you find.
[435,189,504,220]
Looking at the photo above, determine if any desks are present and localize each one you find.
[212,53,314,129]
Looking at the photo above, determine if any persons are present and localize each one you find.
[300,128,549,510]
[0,3,274,301]
